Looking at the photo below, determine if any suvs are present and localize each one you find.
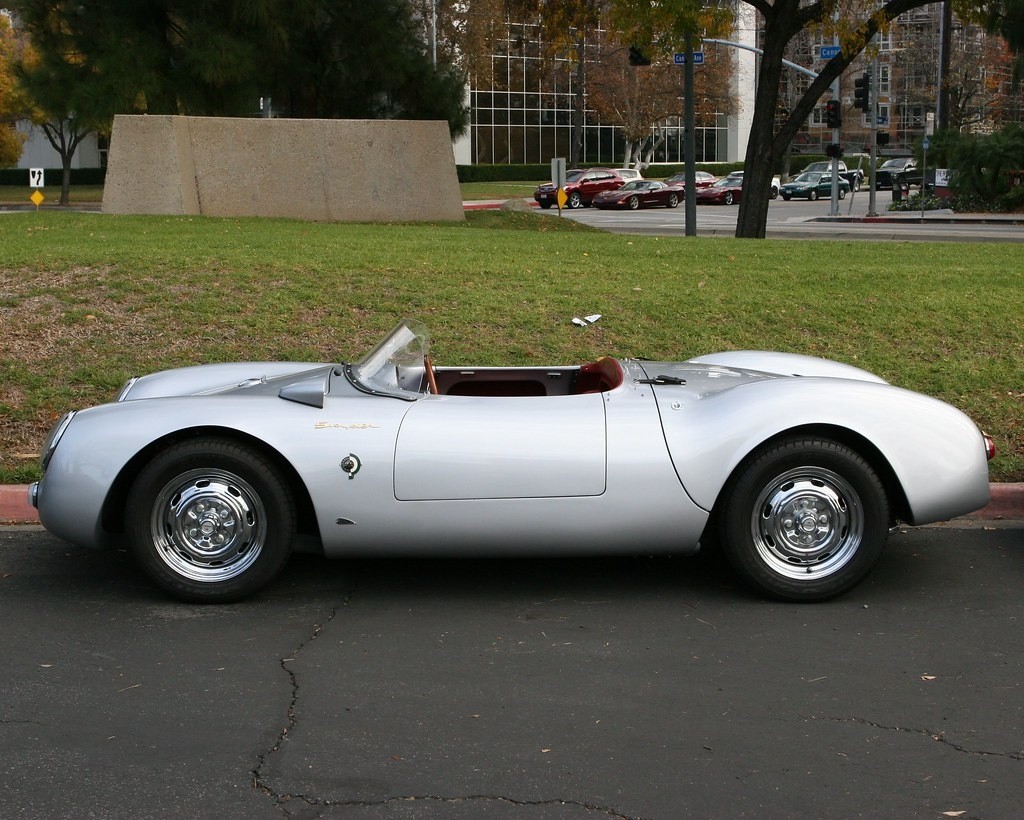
[868,157,923,191]
[611,169,644,182]
[534,167,625,209]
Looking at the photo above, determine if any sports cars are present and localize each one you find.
[696,178,745,206]
[27,329,997,605]
[591,180,685,210]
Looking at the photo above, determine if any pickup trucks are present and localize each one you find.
[792,161,866,193]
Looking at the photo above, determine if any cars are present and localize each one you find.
[779,172,851,201]
[662,172,720,192]
[728,171,780,200]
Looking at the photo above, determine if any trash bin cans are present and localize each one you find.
[891,180,910,202]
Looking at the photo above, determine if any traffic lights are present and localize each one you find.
[854,72,870,113]
[628,31,651,67]
[827,100,841,128]
[834,143,844,159]
[826,145,837,157]
